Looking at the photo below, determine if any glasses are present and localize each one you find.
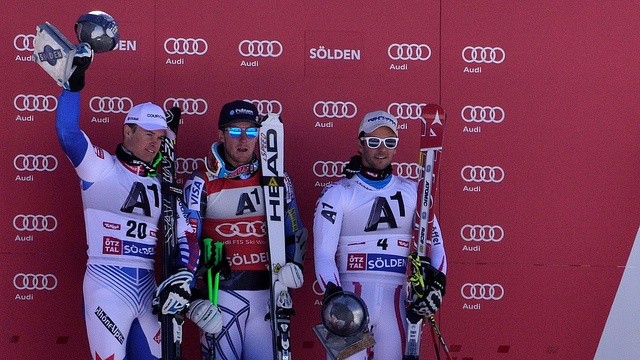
[227,125,259,139]
[359,136,399,150]
[205,141,225,179]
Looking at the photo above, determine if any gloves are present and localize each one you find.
[278,262,306,290]
[408,265,447,320]
[187,298,223,335]
[151,271,196,321]
[63,42,95,92]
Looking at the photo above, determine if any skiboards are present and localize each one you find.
[163,106,182,359]
[259,113,294,360]
[402,102,447,359]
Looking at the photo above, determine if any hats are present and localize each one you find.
[123,101,177,141]
[359,110,398,138]
[217,100,263,130]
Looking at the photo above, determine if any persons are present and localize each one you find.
[55,43,180,359]
[313,110,447,360]
[184,99,304,360]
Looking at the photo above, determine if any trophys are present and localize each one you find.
[30,11,119,87]
[313,277,376,360]
